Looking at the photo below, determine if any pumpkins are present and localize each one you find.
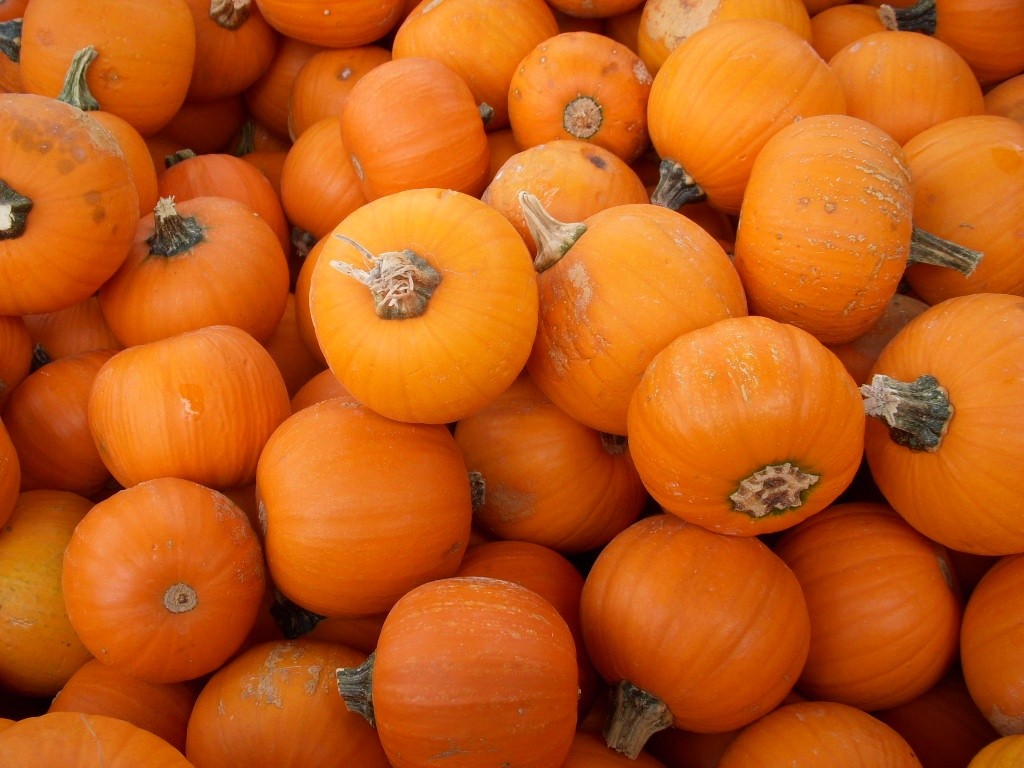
[0,0,1024,768]
